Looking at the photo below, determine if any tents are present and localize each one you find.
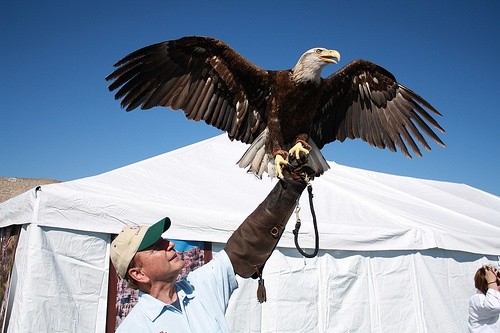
[0,133,500,332]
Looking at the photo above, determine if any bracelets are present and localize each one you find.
[487,281,496,285]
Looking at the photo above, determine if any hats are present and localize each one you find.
[110,215,172,279]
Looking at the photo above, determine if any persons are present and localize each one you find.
[467,264,500,333]
[109,146,316,333]
